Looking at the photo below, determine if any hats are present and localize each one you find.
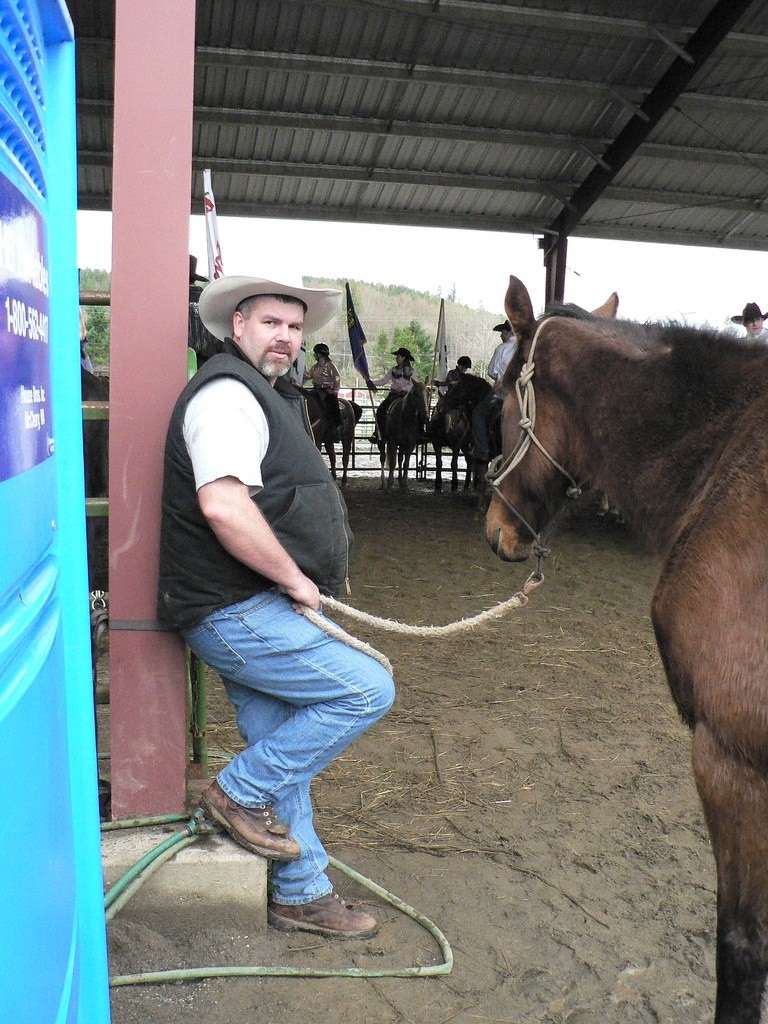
[197,263,343,343]
[731,303,768,323]
[189,255,209,283]
[392,348,416,362]
[493,320,512,331]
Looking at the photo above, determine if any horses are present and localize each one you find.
[81,361,110,617]
[377,375,430,496]
[478,274,768,1024]
[290,384,363,486]
[428,366,503,493]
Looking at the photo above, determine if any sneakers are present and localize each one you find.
[268,890,378,939]
[199,779,301,861]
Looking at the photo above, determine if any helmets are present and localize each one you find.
[459,356,471,368]
[313,344,329,355]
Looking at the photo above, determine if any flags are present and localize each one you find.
[345,285,370,380]
[291,340,308,386]
[203,177,223,282]
[436,302,448,397]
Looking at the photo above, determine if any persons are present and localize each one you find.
[431,357,471,444]
[158,272,396,941]
[731,302,768,345]
[303,344,343,442]
[367,348,432,444]
[188,255,226,367]
[467,321,519,462]
